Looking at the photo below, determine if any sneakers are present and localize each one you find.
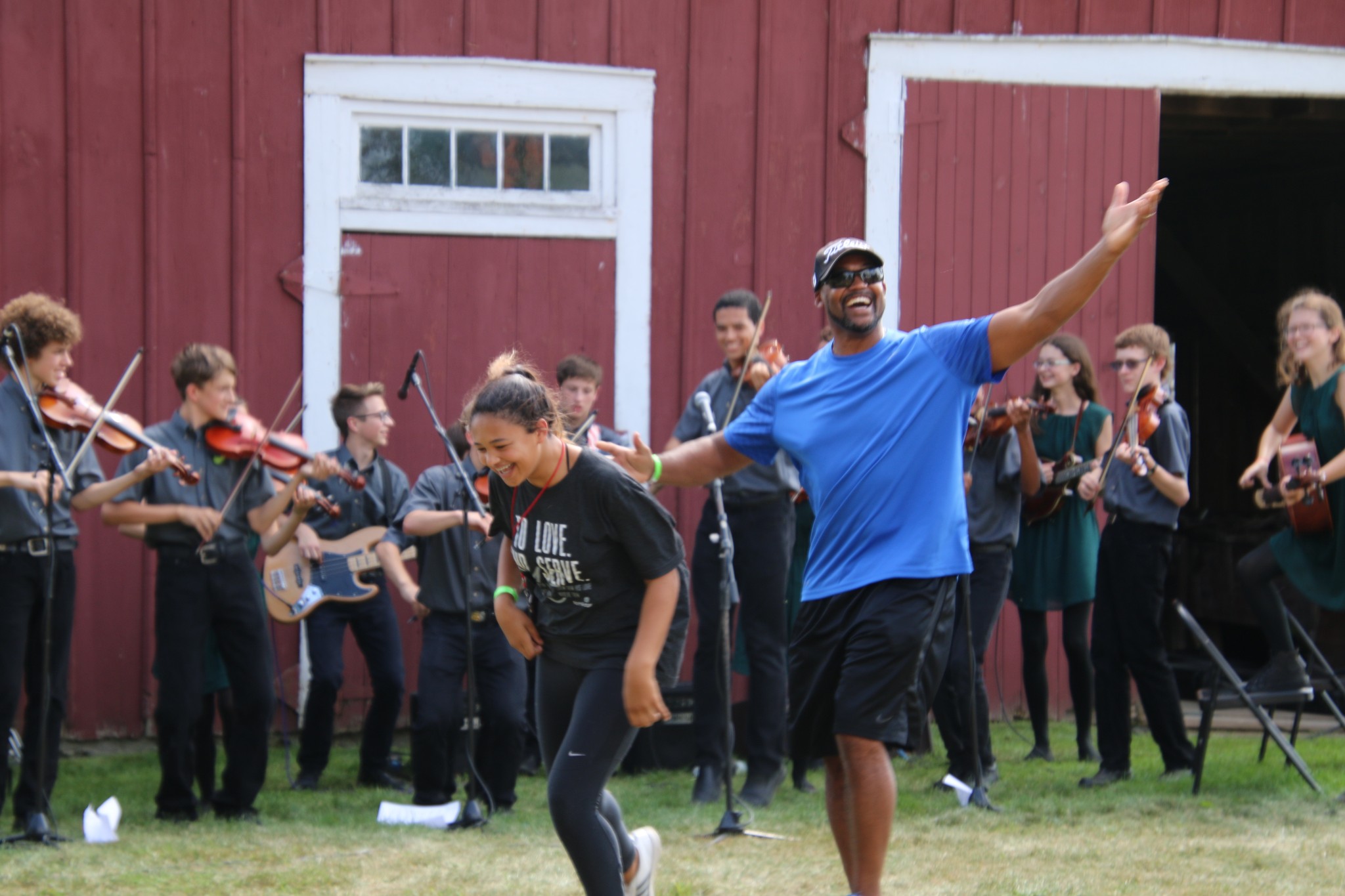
[622,825,661,896]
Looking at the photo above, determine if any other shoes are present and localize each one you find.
[1022,742,1057,762]
[1078,744,1104,763]
[286,769,322,792]
[355,771,409,790]
[11,811,72,842]
[1242,644,1311,693]
[1079,768,1132,787]
[1158,765,1193,785]
[932,765,1003,792]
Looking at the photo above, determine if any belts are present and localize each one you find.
[163,542,248,566]
[439,609,496,622]
[0,536,78,556]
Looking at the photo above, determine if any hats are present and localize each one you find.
[813,236,884,291]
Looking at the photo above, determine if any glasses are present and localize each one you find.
[820,263,883,289]
[355,410,393,421]
[1284,323,1327,339]
[1111,358,1149,370]
[1033,360,1073,369]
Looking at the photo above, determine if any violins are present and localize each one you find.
[731,337,782,385]
[206,414,366,491]
[1125,381,1166,477]
[37,378,200,486]
[984,397,1057,436]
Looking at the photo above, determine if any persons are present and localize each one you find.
[275,381,416,791]
[1005,332,1114,768]
[465,350,693,896]
[925,379,1039,797]
[1,291,178,847]
[374,424,544,818]
[1076,322,1197,793]
[596,174,1170,896]
[1237,290,1345,694]
[662,289,837,810]
[552,351,632,458]
[100,343,341,824]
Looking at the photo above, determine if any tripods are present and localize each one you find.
[0,353,78,851]
[686,429,798,843]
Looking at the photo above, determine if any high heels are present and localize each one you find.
[737,760,788,811]
[691,754,723,807]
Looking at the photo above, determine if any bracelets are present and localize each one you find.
[493,584,519,603]
[649,454,663,486]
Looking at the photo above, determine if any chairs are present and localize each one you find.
[1192,589,1314,796]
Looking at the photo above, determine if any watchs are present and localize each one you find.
[1147,462,1158,478]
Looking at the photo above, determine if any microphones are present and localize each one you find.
[694,391,716,431]
[0,325,13,352]
[398,350,421,398]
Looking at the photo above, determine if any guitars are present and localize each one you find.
[263,521,419,624]
[1277,432,1334,537]
[1019,450,1103,527]
